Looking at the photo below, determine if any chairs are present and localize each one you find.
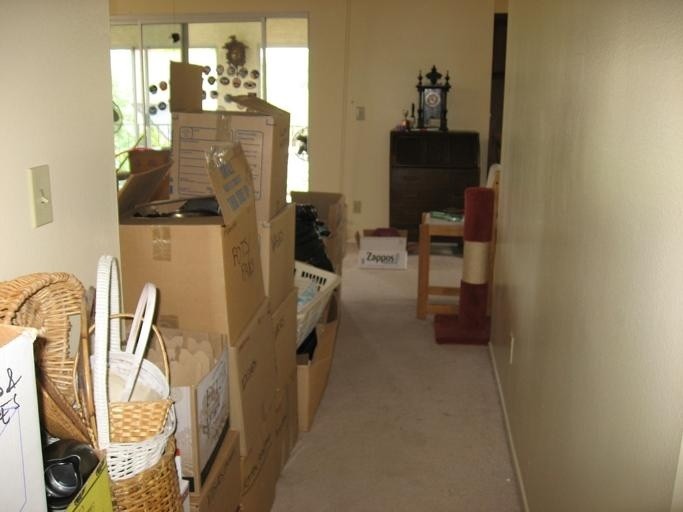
[415,162,503,322]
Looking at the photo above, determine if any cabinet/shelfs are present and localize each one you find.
[389,129,481,254]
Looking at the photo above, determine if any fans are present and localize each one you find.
[112,101,125,136]
[290,126,308,162]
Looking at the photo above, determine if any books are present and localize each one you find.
[411,128,440,132]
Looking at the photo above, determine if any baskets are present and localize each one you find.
[109,433,184,512]
[89,282,170,403]
[293,260,341,351]
[92,255,178,481]
[0,272,94,449]
[72,313,176,443]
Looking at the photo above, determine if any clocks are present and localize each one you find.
[222,35,249,74]
[415,64,450,131]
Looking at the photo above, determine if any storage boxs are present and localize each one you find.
[352,225,409,271]
[165,60,296,227]
[0,321,53,512]
[54,447,114,512]
